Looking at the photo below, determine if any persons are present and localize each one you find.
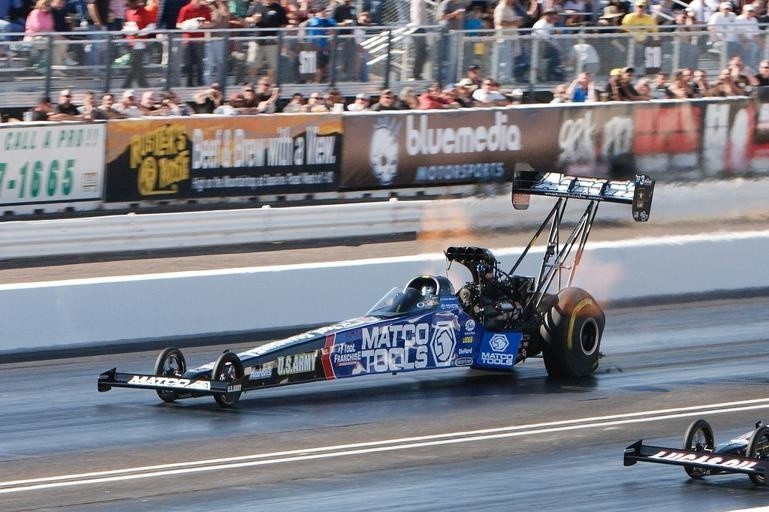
[419,283,436,297]
[0,1,769,124]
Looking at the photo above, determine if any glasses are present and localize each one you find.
[31,0,769,111]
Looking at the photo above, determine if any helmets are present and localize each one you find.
[401,276,440,303]
